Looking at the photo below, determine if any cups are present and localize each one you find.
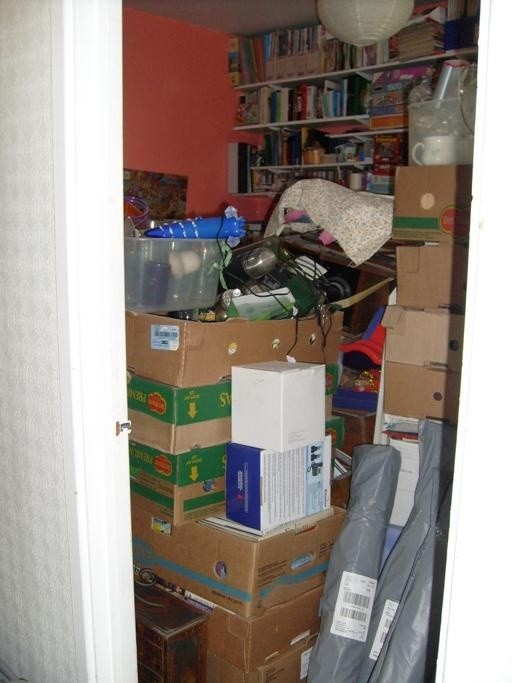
[142,259,169,307]
[349,172,362,190]
[124,197,152,231]
[412,134,459,168]
[168,249,203,281]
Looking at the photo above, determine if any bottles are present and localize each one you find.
[217,249,289,291]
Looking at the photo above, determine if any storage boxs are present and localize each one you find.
[125,165,471,681]
[405,96,475,168]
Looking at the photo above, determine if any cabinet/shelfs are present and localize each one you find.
[232,44,477,199]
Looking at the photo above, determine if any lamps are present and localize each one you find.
[315,0,416,45]
